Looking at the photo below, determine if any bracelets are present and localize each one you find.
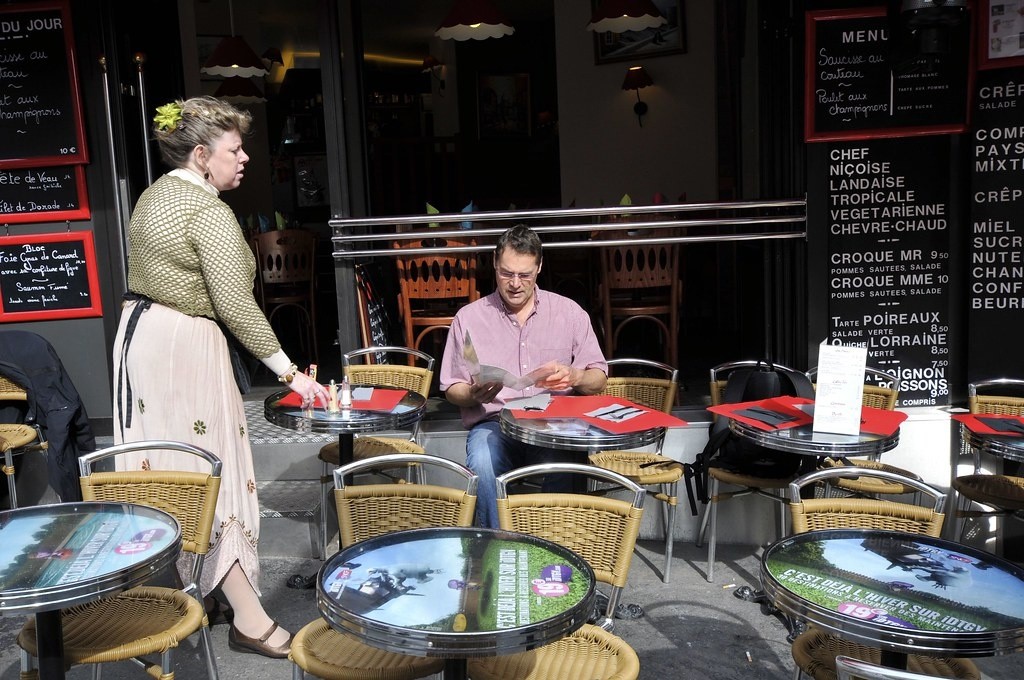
[279,366,298,382]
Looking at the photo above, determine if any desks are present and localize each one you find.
[501,396,668,621]
[959,425,1024,569]
[759,528,1024,675]
[728,417,900,638]
[316,527,596,680]
[264,383,427,589]
[0,501,183,680]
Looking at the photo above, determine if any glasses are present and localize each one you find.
[496,256,539,280]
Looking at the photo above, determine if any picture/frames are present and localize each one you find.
[591,0,688,66]
[290,152,330,211]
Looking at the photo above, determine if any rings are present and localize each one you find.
[304,398,309,400]
[315,390,320,394]
[311,396,315,399]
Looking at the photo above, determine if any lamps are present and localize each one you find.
[585,0,668,34]
[620,65,654,115]
[199,0,271,77]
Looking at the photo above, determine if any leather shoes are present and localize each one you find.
[227,616,295,658]
[201,595,233,625]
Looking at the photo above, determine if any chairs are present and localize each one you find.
[0,196,1024,680]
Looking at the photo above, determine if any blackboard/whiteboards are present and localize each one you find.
[0,167,91,226]
[0,0,90,169]
[806,4,968,140]
[0,233,103,322]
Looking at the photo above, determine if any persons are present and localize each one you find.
[439,223,608,529]
[112,95,332,659]
